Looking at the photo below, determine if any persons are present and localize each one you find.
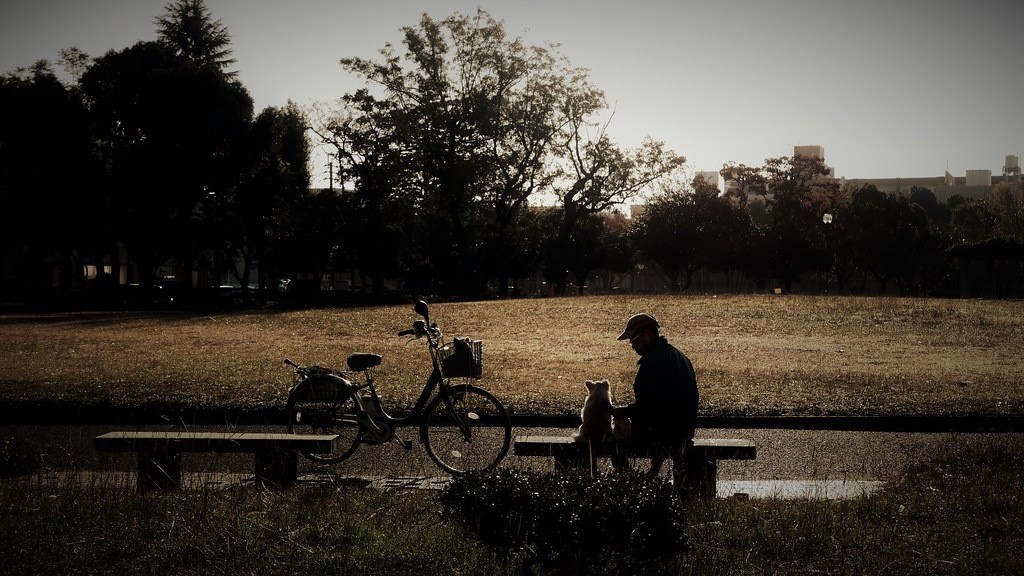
[612,314,699,476]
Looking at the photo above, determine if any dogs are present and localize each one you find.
[443,338,473,368]
[572,380,632,443]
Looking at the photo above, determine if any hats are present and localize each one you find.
[616,312,659,341]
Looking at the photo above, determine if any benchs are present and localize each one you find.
[97,431,340,485]
[514,435,757,500]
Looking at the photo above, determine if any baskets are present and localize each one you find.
[436,338,484,378]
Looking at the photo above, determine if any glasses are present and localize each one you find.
[628,332,642,345]
